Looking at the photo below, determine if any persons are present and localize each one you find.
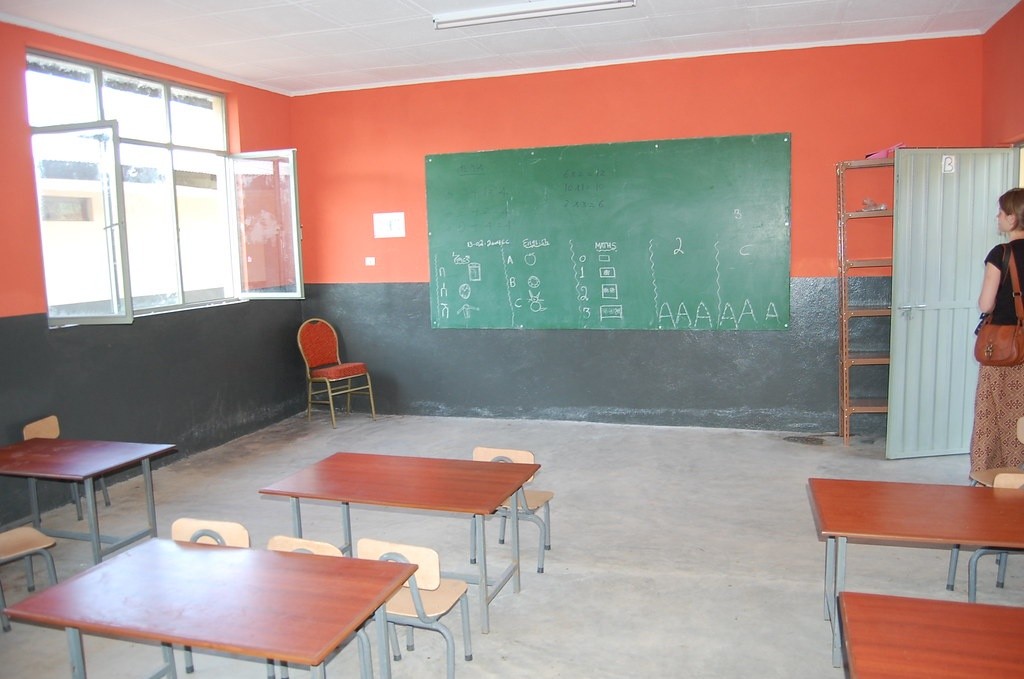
[968,188,1024,484]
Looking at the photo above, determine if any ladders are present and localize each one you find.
[835,154,894,446]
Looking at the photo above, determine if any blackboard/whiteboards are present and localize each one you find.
[424,131,793,334]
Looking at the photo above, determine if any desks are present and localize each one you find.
[808,477,1024,667]
[837,591,1024,679]
[5,537,418,679]
[259,451,541,633]
[0,437,176,565]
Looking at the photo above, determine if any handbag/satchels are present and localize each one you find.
[974,324,1024,367]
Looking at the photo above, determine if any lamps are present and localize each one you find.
[432,0,636,30]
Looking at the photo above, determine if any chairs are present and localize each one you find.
[969,416,1024,564]
[946,473,1024,604]
[170,517,274,679]
[23,416,111,521]
[0,526,58,632]
[297,319,376,429]
[355,538,473,679]
[268,536,402,679]
[470,446,554,574]
